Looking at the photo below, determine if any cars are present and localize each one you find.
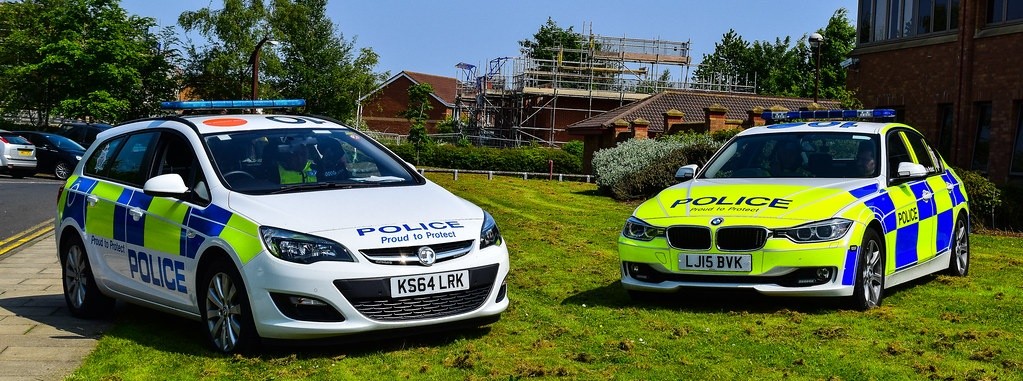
[0,129,40,177]
[618,109,972,312]
[53,100,509,356]
[57,122,149,171]
[13,131,87,180]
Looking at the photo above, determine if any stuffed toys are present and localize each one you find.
[310,138,353,182]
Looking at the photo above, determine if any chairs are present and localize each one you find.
[806,152,832,176]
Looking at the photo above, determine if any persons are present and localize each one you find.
[844,140,877,177]
[762,140,813,176]
[273,144,319,183]
